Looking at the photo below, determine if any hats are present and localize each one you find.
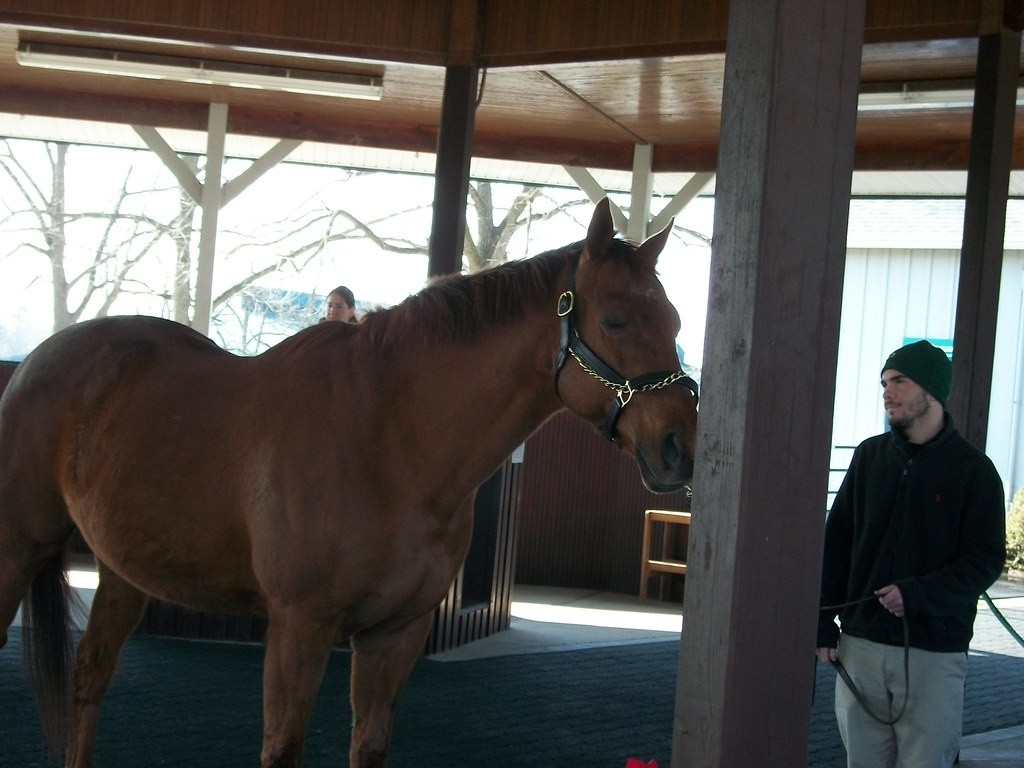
[882,339,955,404]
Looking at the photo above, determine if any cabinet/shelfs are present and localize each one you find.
[638,509,691,605]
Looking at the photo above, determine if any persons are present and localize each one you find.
[316,284,358,325]
[814,339,1005,768]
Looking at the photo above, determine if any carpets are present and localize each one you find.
[0,626,1024,768]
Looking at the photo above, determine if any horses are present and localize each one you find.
[1,196,698,768]
